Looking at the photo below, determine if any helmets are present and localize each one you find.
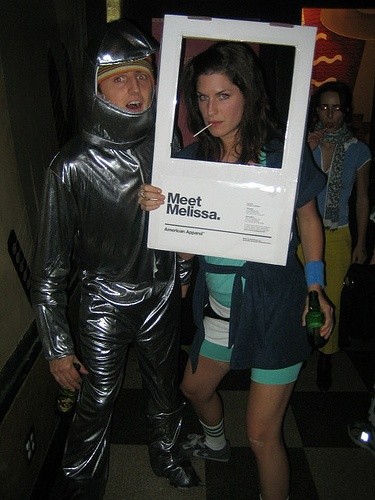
[80,21,159,149]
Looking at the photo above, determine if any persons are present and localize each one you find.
[32,18,375,500]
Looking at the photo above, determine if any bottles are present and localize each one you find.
[57,363,81,416]
[305,290,330,348]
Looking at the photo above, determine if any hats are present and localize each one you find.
[98,55,155,86]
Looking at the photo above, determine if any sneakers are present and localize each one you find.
[178,433,229,461]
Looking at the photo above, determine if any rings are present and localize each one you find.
[141,191,146,199]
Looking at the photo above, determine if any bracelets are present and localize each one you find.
[305,260,325,289]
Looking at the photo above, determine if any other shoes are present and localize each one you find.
[317,353,333,390]
[347,420,375,458]
